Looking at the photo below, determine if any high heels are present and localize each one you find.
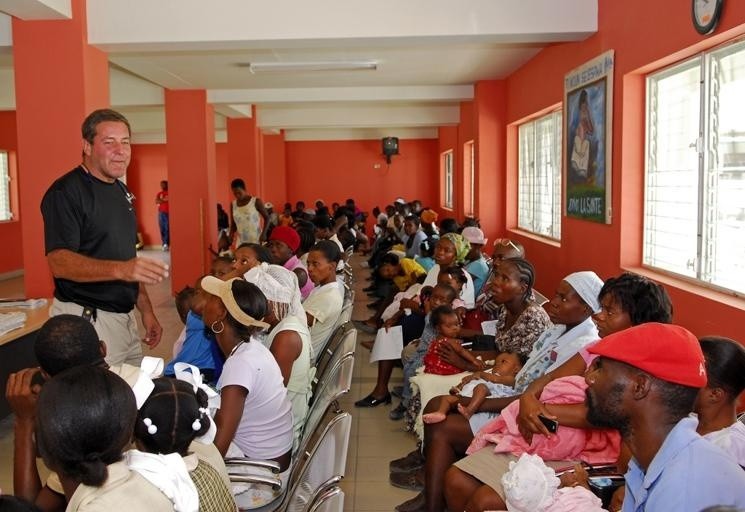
[353,391,392,408]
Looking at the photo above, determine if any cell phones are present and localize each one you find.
[537,414,559,434]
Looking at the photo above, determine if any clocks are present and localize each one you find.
[689,0,724,36]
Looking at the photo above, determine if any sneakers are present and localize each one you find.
[389,465,427,491]
[161,242,169,252]
[353,318,378,336]
[388,441,425,472]
[389,401,408,421]
[359,260,378,310]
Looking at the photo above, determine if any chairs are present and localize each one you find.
[222,244,627,511]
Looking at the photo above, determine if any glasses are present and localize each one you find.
[493,237,521,253]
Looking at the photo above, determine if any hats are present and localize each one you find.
[584,319,710,391]
[561,268,607,316]
[393,197,406,205]
[269,225,301,254]
[460,226,489,246]
[264,201,274,209]
[200,274,272,334]
[420,208,439,225]
[499,452,561,512]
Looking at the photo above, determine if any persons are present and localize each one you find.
[227,178,268,250]
[568,91,601,188]
[41,109,172,372]
[155,180,170,251]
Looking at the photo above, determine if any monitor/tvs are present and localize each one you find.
[382,137,398,154]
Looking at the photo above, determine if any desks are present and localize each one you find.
[0,298,55,418]
